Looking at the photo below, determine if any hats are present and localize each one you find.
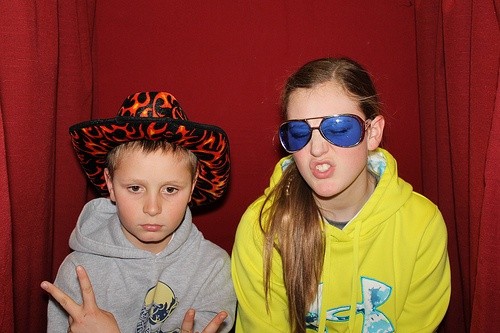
[66,91,231,215]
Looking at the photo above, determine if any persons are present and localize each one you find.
[231,57,451,333]
[40,92,237,333]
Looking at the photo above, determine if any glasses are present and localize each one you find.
[277,113,372,154]
